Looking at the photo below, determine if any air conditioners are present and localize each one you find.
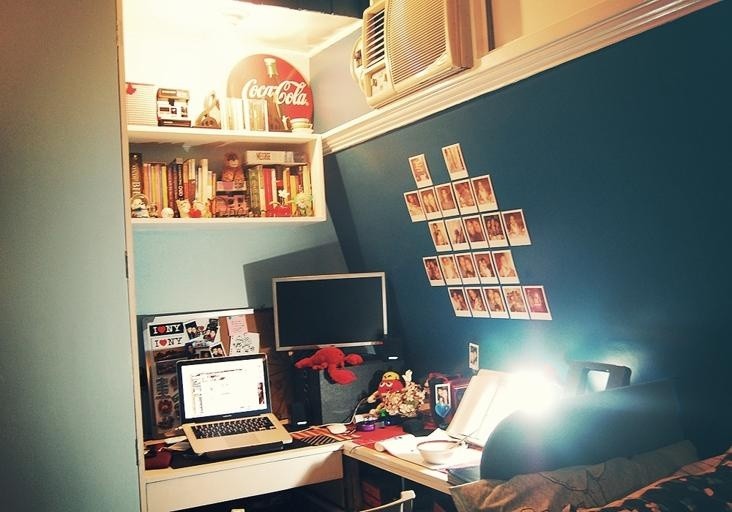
[350,0,490,109]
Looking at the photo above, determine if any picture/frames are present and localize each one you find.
[468,342,479,371]
[434,384,451,409]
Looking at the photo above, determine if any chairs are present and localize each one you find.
[231,488,415,511]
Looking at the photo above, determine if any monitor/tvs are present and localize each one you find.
[271,271,389,367]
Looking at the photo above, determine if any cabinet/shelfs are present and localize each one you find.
[128,124,326,223]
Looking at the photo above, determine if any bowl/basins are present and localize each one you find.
[417,438,468,465]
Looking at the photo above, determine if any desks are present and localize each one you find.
[142,421,342,512]
[344,413,482,496]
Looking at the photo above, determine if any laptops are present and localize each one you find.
[175,353,294,459]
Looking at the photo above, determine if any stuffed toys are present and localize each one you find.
[365,369,416,418]
[293,346,365,386]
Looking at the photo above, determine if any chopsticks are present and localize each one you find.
[445,431,479,451]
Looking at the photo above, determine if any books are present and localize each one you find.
[129,149,315,217]
[443,465,482,487]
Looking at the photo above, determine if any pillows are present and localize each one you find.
[565,447,731,512]
[449,440,699,512]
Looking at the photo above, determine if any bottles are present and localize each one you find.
[261,57,291,131]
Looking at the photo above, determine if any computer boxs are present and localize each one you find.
[293,342,405,425]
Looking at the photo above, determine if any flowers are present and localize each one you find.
[383,381,425,417]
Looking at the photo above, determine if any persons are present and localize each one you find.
[434,385,449,407]
[470,346,477,365]
[402,144,548,316]
[183,319,225,357]
